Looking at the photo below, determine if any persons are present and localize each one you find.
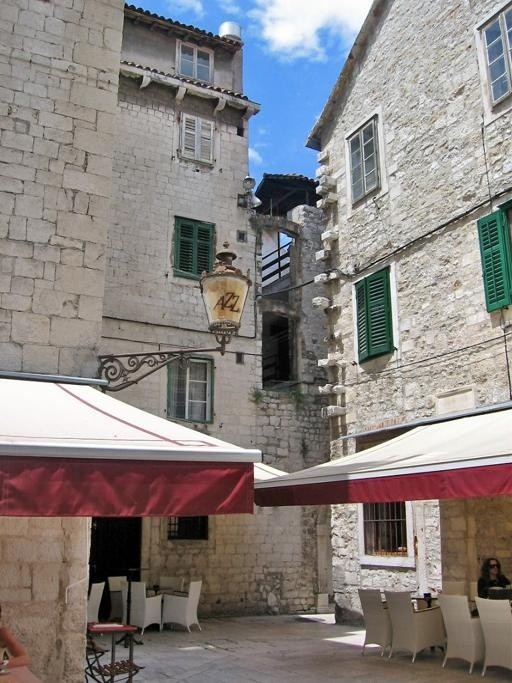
[0,606,31,669]
[476,556,510,604]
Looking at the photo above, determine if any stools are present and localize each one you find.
[86,641,146,683]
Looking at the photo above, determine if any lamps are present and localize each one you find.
[98,244,253,393]
[237,175,261,210]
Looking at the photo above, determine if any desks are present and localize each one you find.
[85,619,144,683]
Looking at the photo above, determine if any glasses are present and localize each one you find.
[489,564,500,568]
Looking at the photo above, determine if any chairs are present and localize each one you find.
[87,576,203,636]
[357,589,512,677]
